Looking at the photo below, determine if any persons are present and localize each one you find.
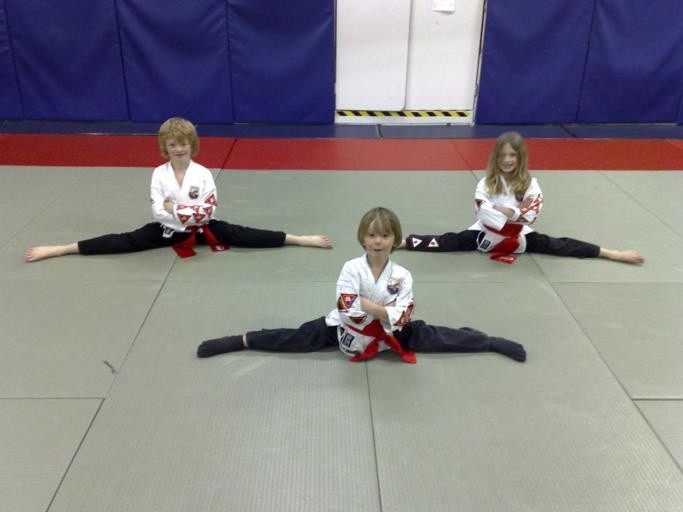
[25,115,333,263]
[396,129,644,266]
[196,205,528,363]
